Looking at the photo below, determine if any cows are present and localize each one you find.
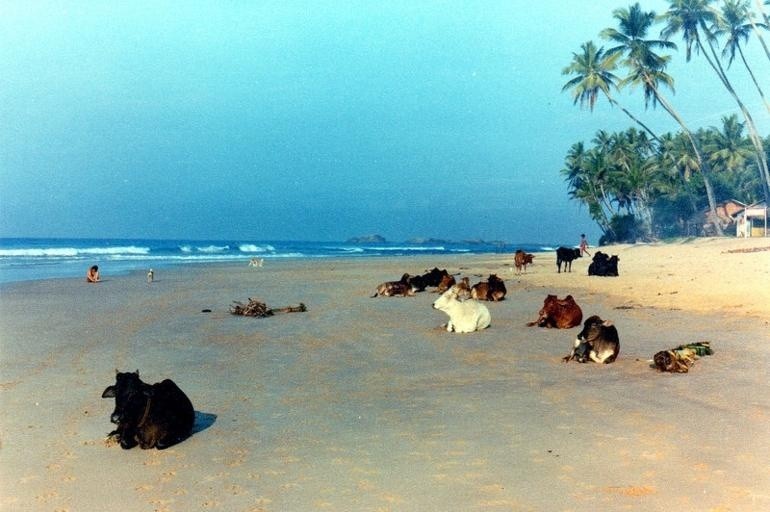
[514,249,535,274]
[555,246,581,272]
[99,367,196,451]
[588,251,621,278]
[367,266,507,334]
[525,292,583,331]
[561,313,622,366]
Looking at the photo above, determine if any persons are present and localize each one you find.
[579,234,591,257]
[86,265,101,284]
[738,216,745,238]
